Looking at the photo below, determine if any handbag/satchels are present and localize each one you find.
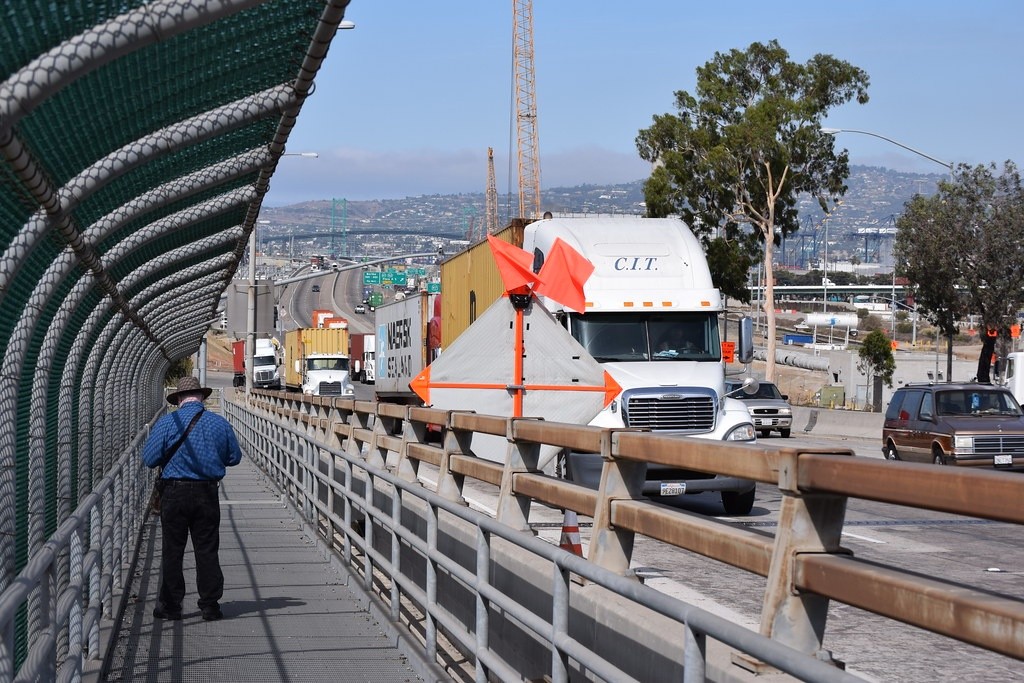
[150,478,161,515]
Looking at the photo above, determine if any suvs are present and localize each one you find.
[723,379,793,439]
[880,381,1024,473]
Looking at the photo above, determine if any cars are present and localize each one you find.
[354,304,365,314]
[312,285,320,292]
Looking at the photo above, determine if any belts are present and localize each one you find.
[161,479,218,488]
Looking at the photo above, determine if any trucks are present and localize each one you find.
[323,317,348,333]
[285,327,356,402]
[368,292,383,312]
[362,287,374,304]
[349,332,376,385]
[440,205,762,518]
[991,350,1024,416]
[311,309,333,329]
[372,290,441,443]
[231,337,281,390]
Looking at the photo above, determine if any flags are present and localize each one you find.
[487,234,546,292]
[534,238,594,314]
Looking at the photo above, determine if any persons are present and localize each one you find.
[660,327,705,354]
[979,397,990,410]
[143,377,244,618]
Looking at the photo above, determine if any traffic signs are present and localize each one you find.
[363,267,426,287]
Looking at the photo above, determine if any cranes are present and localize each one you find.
[485,146,498,239]
[510,2,542,221]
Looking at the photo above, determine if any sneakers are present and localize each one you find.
[154,604,182,620]
[202,606,223,620]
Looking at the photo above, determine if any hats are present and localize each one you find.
[166,376,212,405]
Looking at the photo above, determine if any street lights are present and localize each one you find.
[819,126,954,384]
